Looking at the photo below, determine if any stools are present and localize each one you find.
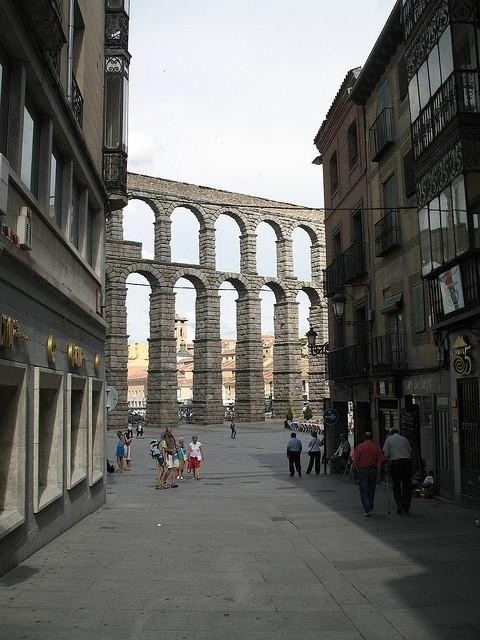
[338,456,354,482]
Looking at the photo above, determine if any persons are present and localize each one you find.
[230,422,236,439]
[136,425,144,439]
[383,427,418,516]
[329,433,351,466]
[155,427,204,490]
[286,432,302,478]
[305,431,324,475]
[349,431,381,517]
[116,423,132,471]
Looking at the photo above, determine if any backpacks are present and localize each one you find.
[149,439,164,457]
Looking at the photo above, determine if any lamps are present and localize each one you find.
[326,283,376,335]
[303,325,331,357]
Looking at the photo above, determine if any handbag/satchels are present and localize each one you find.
[184,455,188,461]
